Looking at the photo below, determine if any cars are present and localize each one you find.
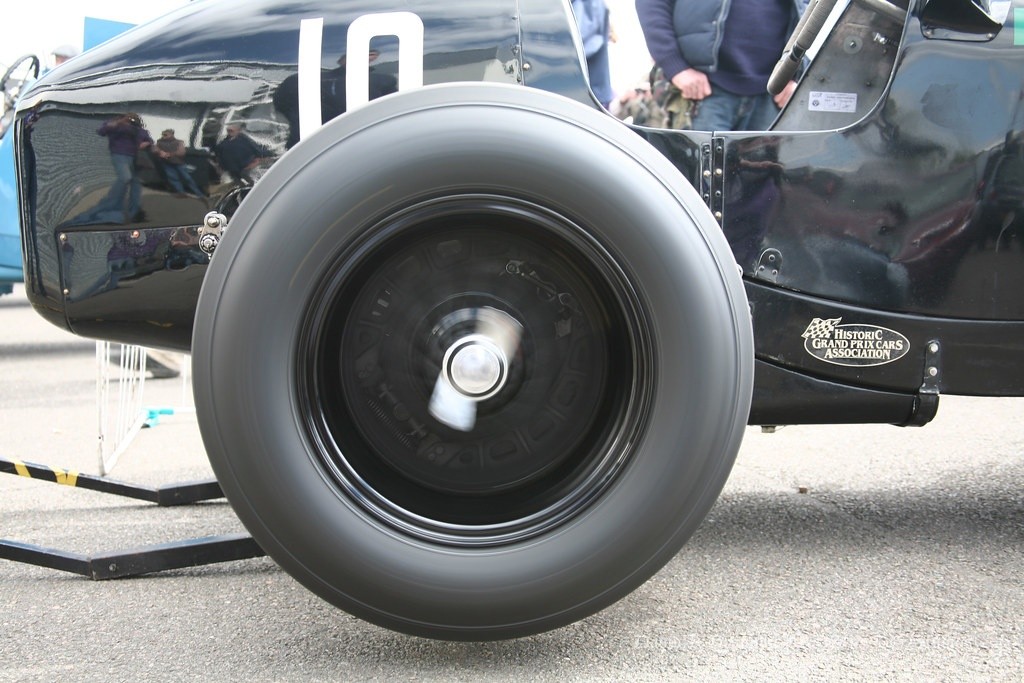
[0,0,1024,646]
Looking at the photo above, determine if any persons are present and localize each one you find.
[612,82,665,129]
[570,0,614,111]
[66,112,259,291]
[635,0,810,130]
[275,48,397,151]
